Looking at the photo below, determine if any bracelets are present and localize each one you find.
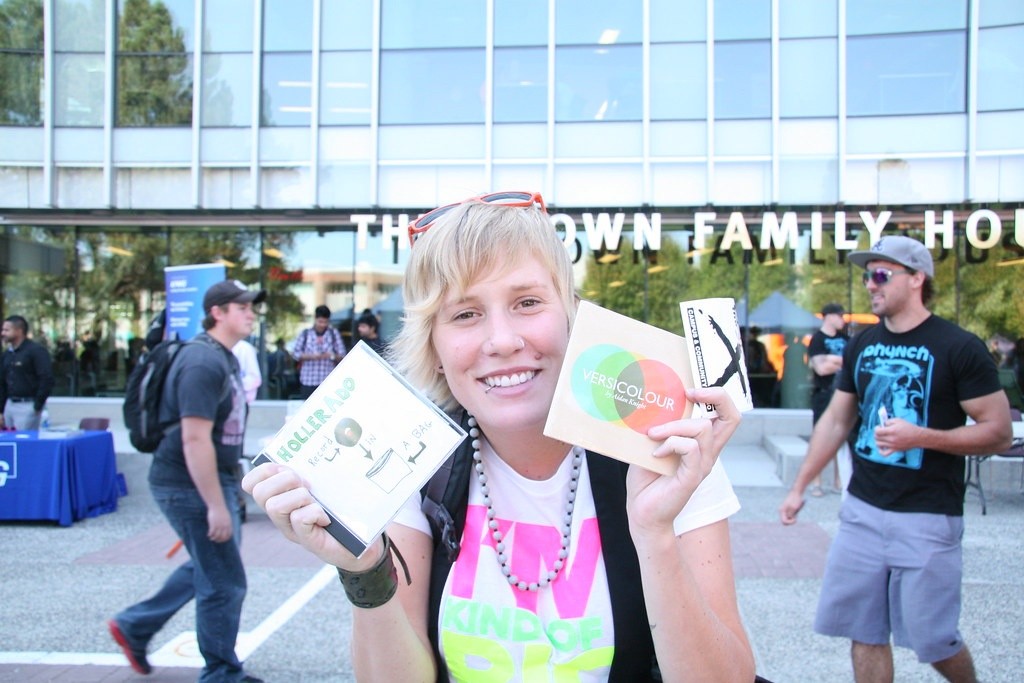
[331,355,335,360]
[337,531,398,608]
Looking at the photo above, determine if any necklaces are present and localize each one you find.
[467,412,581,592]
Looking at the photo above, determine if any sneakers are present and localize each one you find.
[107,618,152,676]
[240,676,266,683]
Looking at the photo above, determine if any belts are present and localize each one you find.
[7,396,38,404]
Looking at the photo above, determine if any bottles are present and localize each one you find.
[39,407,50,431]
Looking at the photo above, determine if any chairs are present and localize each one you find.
[963,409,1024,515]
[79,417,110,432]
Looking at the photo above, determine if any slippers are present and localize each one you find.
[829,485,846,495]
[810,485,825,498]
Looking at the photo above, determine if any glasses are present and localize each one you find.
[862,267,909,286]
[408,190,547,247]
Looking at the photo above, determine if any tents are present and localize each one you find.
[750,290,822,334]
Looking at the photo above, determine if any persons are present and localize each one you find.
[808,303,851,498]
[990,339,1001,363]
[748,327,808,408]
[779,236,1013,683]
[294,305,387,404]
[271,338,290,369]
[0,316,56,431]
[108,279,267,683]
[240,191,756,683]
[80,331,100,392]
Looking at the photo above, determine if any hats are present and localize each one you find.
[848,235,934,280]
[822,304,852,315]
[202,279,268,315]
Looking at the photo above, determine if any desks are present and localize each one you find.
[0,428,128,526]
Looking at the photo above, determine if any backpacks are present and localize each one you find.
[121,332,232,454]
